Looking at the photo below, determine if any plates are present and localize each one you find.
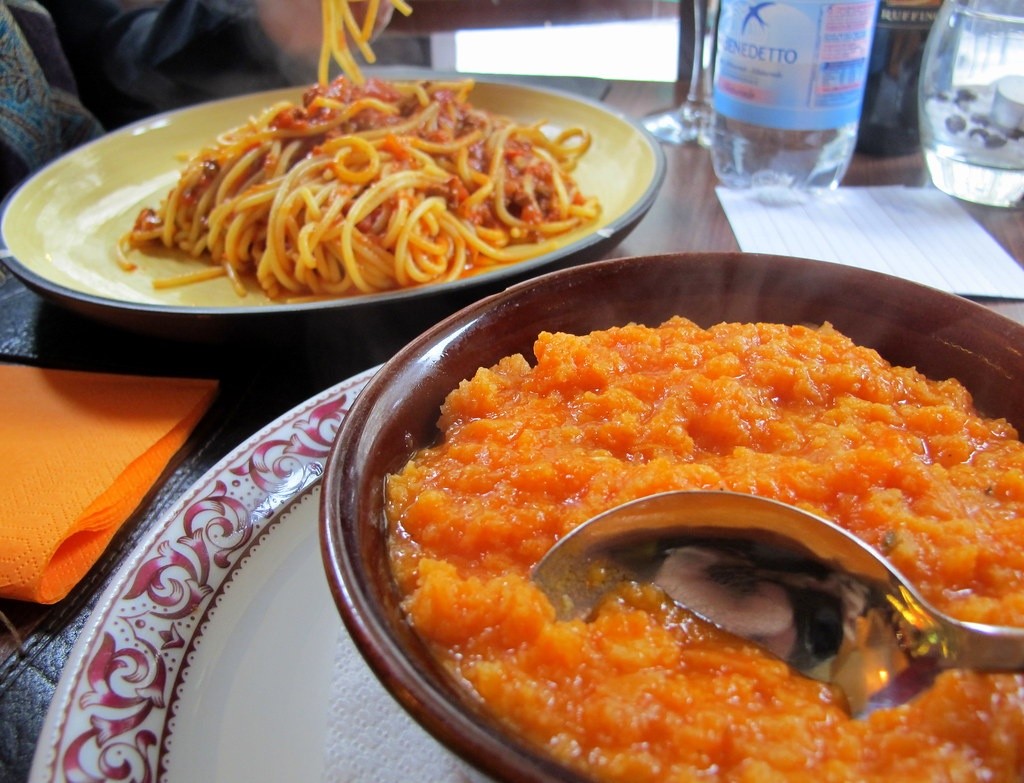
[30,364,495,783]
[0,82,667,333]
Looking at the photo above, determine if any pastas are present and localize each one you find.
[115,0,600,305]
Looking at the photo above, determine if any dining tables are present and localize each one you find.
[0,69,1024,783]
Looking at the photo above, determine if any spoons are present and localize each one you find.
[529,490,1024,721]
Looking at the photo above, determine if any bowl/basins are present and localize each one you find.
[318,253,1024,783]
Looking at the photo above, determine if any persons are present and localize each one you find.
[0,0,396,201]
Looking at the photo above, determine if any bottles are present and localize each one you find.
[857,0,941,158]
[709,1,877,204]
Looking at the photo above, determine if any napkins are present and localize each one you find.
[0,362,218,606]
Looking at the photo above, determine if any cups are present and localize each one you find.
[917,2,1024,209]
